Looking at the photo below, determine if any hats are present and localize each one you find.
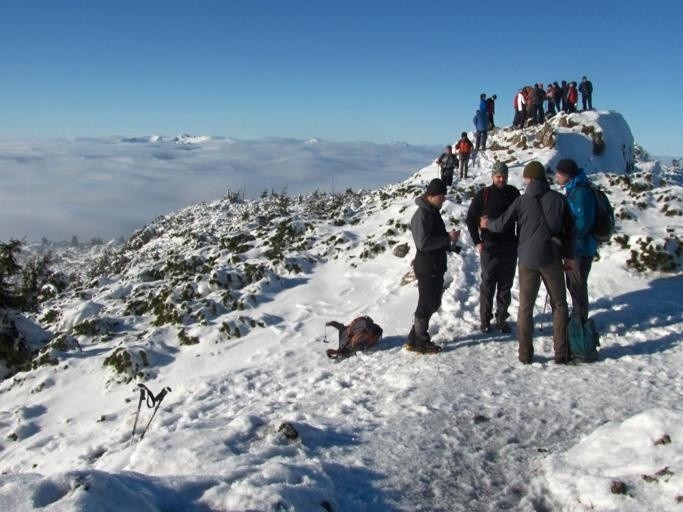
[524,161,546,179]
[556,159,579,176]
[425,178,447,195]
[491,164,508,177]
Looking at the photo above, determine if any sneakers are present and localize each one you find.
[405,338,416,351]
[518,353,532,365]
[498,319,510,332]
[480,321,489,330]
[557,354,576,365]
[415,341,440,354]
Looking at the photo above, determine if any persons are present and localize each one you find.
[481,160,568,363]
[456,132,474,178]
[437,145,459,186]
[474,110,488,150]
[486,95,497,126]
[480,94,487,114]
[579,77,593,110]
[556,159,598,321]
[408,178,460,354]
[467,162,521,332]
[513,80,577,129]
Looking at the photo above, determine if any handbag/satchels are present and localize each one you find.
[566,316,600,363]
[537,197,573,262]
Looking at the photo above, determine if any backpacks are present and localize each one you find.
[571,182,615,241]
[323,314,382,359]
